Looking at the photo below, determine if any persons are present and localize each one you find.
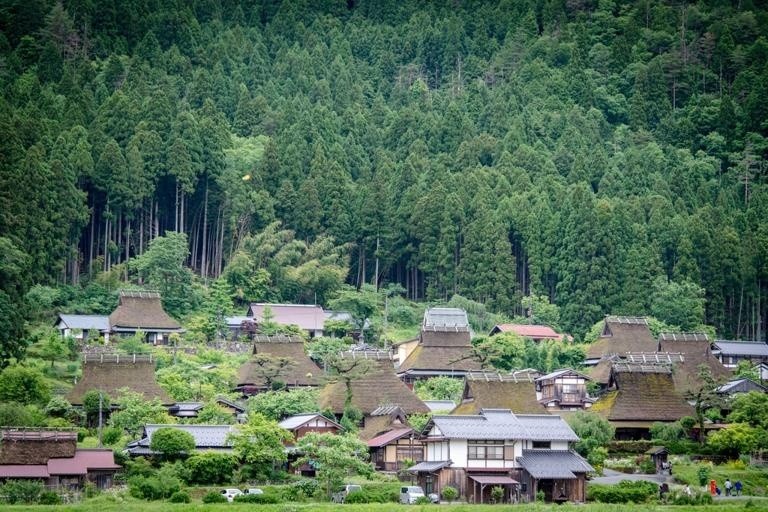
[660,483,666,498]
[669,462,672,475]
[685,485,690,496]
[735,481,741,496]
[637,455,640,465]
[725,479,732,496]
[662,460,667,470]
[716,488,721,494]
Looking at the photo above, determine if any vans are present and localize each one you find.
[331,483,362,503]
[400,485,425,504]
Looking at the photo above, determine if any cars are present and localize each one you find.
[243,488,263,494]
[219,489,244,502]
